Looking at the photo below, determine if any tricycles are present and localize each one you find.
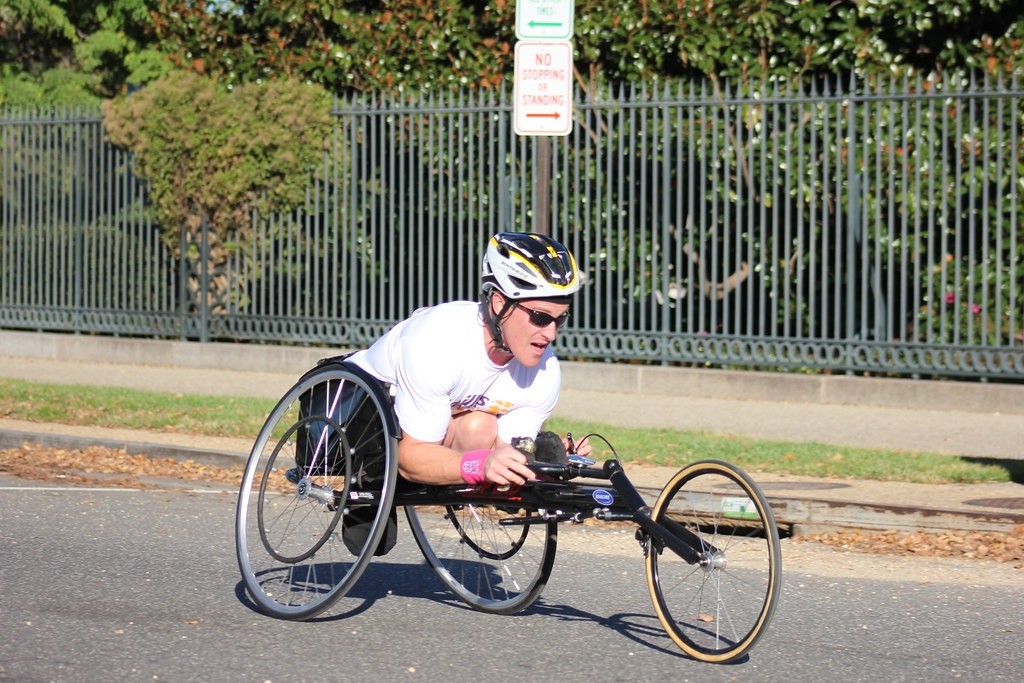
[234,347,800,665]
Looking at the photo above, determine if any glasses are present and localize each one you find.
[517,303,569,327]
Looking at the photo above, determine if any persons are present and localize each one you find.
[308,231,594,501]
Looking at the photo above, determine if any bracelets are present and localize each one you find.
[460,449,494,486]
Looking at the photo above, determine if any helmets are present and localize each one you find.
[481,231,580,299]
